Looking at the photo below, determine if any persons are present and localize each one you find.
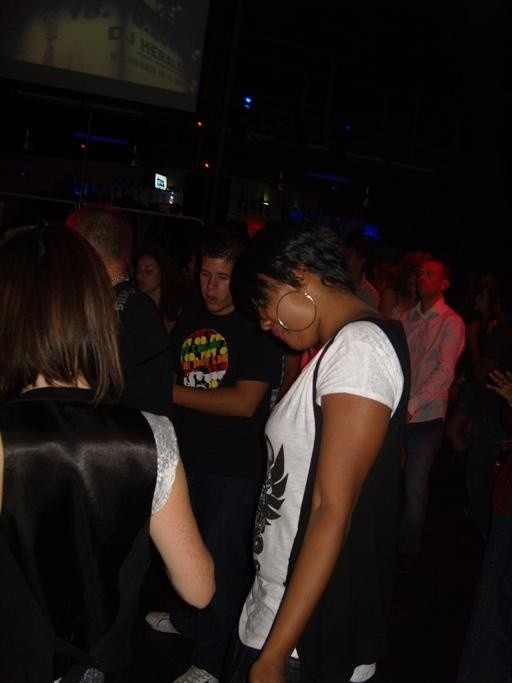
[392,258,466,576]
[380,252,433,321]
[339,235,379,307]
[146,237,287,683]
[443,271,487,328]
[130,247,179,341]
[464,363,511,683]
[216,221,413,683]
[57,202,184,417]
[0,224,216,683]
